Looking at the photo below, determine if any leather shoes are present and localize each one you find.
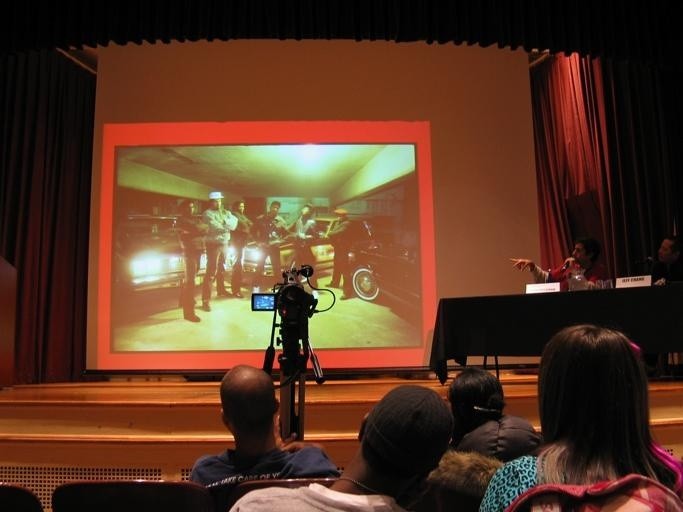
[184,290,242,322]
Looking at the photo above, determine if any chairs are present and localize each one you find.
[0,481,44,511]
[50,479,213,511]
[216,476,337,512]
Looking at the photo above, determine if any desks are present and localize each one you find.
[428,282,683,387]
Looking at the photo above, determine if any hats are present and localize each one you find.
[209,192,224,199]
[334,209,347,215]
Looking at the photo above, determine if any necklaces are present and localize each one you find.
[336,475,379,495]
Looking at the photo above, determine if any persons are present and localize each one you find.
[509,234,610,293]
[187,364,344,485]
[642,235,683,380]
[478,324,682,512]
[448,366,542,456]
[175,191,356,322]
[226,384,456,512]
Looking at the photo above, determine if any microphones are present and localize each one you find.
[645,256,654,265]
[560,257,575,272]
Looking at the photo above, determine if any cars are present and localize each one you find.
[223,215,347,285]
[112,215,208,295]
[347,239,421,309]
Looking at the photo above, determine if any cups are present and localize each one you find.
[596,279,614,289]
